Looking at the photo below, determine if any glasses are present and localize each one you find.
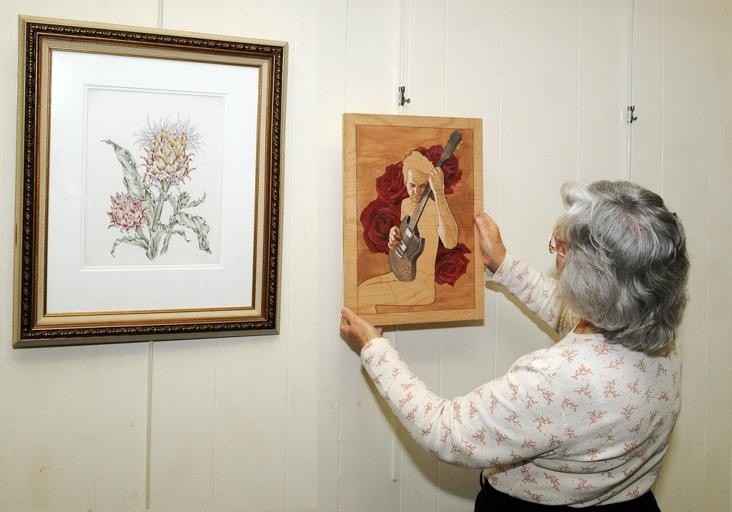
[547,229,565,258]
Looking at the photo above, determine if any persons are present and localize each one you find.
[340,180,689,512]
[357,152,458,315]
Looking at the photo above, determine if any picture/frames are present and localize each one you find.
[342,114,486,327]
[13,14,289,349]
